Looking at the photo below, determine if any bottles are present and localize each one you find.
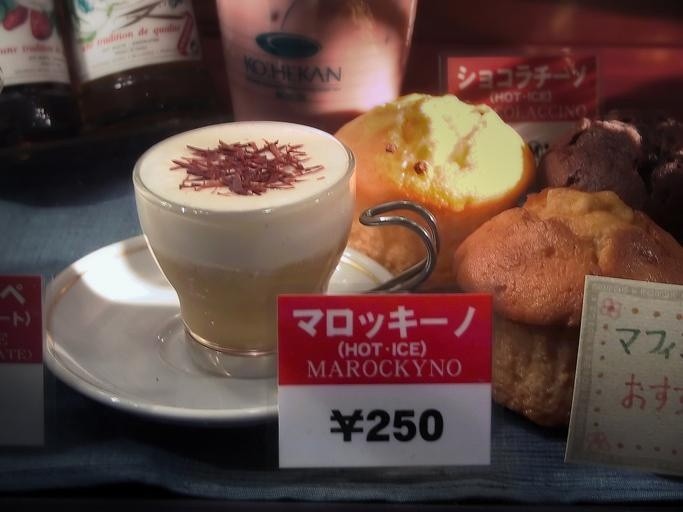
[63,0,214,154]
[217,0,415,137]
[0,0,102,206]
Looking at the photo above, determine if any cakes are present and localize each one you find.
[540,117,642,210]
[643,97,683,217]
[335,93,536,292]
[453,189,683,429]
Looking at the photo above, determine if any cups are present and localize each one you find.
[132,121,356,381]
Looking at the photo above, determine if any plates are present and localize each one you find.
[43,234,410,423]
